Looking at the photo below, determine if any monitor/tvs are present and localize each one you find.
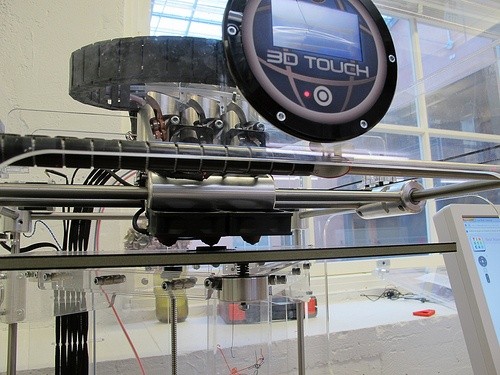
[434,203,500,374]
[272,0,364,63]
